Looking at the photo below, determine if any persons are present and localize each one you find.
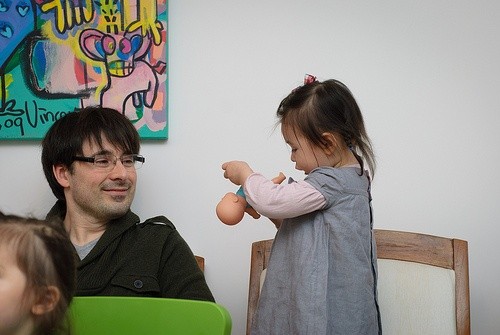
[222,73,383,335]
[41,106,216,304]
[216,172,287,230]
[0,211,80,335]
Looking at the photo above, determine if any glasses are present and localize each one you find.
[73,154,146,170]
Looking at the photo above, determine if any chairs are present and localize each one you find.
[245,229,471,335]
[64,296,232,335]
